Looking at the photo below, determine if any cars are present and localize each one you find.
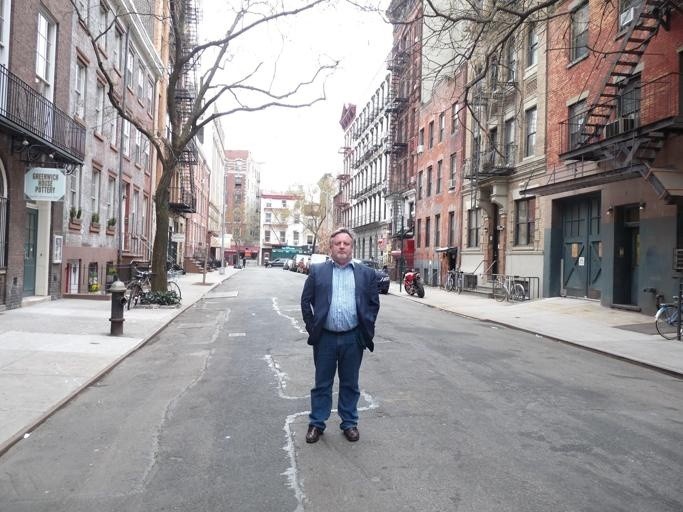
[264,254,330,275]
[353,258,390,294]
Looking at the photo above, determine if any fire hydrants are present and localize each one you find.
[243,257,247,268]
[106,278,127,336]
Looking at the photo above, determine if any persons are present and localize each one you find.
[301,227,380,444]
[264,259,268,268]
[242,257,246,269]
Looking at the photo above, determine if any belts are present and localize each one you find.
[323,329,358,335]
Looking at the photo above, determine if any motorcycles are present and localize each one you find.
[402,268,424,298]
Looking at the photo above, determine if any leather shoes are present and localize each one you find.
[306,425,323,443]
[344,428,359,442]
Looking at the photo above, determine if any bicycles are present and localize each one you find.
[446,271,464,294]
[655,295,683,340]
[494,275,526,302]
[126,264,181,310]
